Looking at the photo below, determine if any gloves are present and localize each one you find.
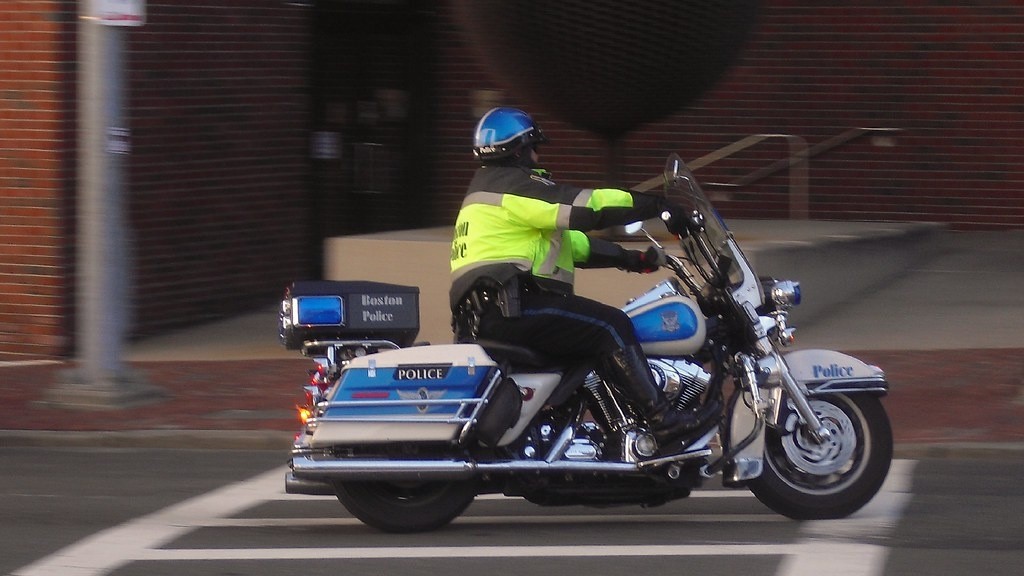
[660,205,703,239]
[642,248,665,272]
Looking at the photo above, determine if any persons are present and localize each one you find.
[449,107,725,458]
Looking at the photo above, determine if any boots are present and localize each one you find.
[608,341,722,445]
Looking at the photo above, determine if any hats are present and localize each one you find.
[472,105,546,158]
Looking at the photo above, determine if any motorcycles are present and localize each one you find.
[280,150,894,532]
